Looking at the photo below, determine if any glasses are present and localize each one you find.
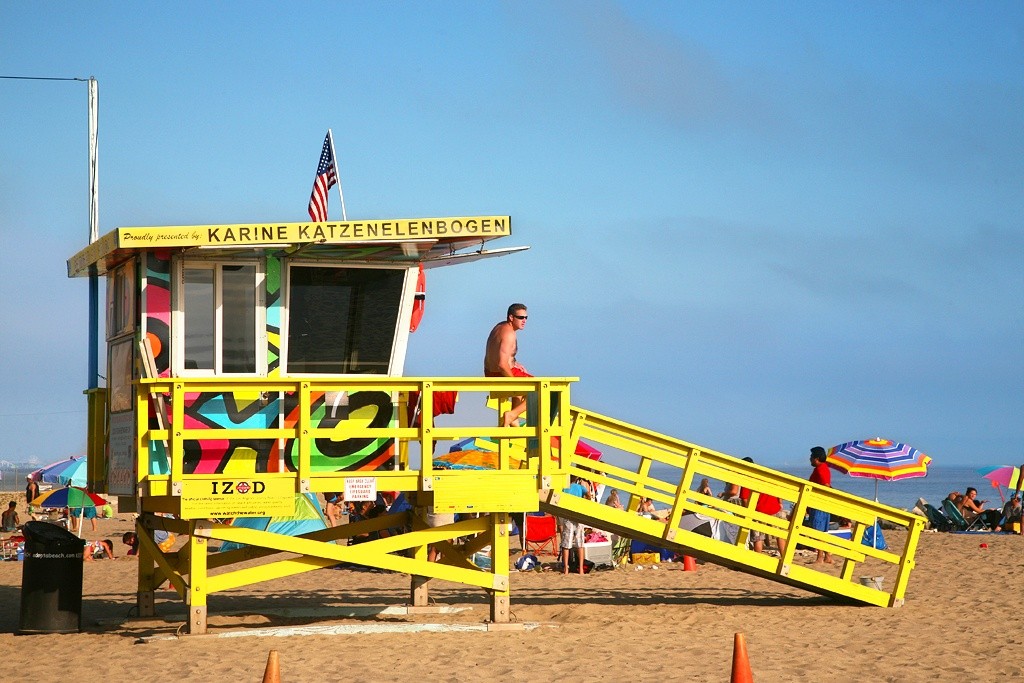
[512,314,528,319]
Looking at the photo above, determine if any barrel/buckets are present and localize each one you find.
[18,543,84,634]
[859,575,884,591]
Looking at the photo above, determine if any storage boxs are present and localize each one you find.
[573,542,612,567]
[632,552,661,565]
[828,529,852,540]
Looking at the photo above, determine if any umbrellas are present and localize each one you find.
[29,485,108,531]
[39,456,87,538]
[489,421,603,471]
[826,437,933,549]
[979,464,1024,513]
[26,451,87,482]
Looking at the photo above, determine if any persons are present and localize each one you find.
[485,303,534,427]
[25,474,40,522]
[84,539,113,561]
[941,487,1022,532]
[740,457,786,559]
[716,482,741,504]
[697,478,712,505]
[324,492,454,563]
[2,501,19,531]
[806,447,834,564]
[639,497,652,513]
[123,532,139,555]
[558,461,620,575]
[70,504,113,532]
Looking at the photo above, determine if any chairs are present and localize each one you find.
[524,516,558,556]
[941,499,989,530]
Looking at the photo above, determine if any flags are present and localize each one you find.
[308,133,337,222]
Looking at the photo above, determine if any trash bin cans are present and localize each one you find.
[17,520,87,633]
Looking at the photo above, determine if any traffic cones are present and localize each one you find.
[263,649,280,683]
[682,554,697,572]
[731,632,754,683]
